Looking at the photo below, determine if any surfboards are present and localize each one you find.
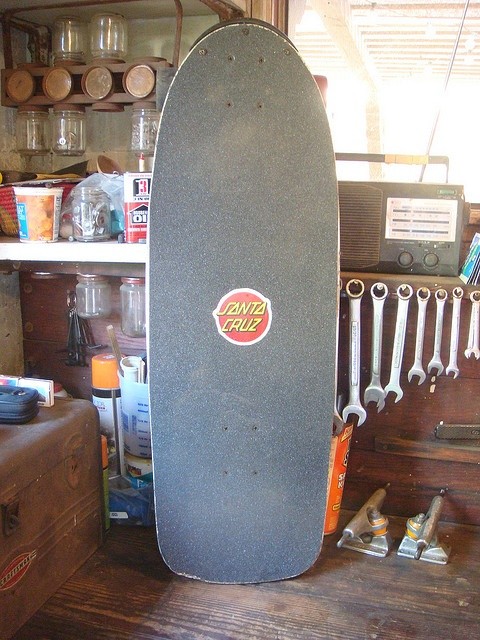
[145,16,341,586]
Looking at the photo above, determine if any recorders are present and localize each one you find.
[335,153,464,277]
